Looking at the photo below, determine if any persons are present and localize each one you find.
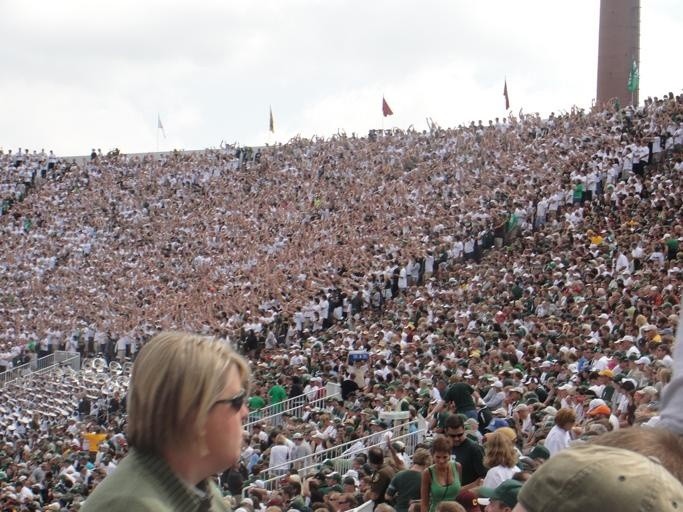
[0,93,682,512]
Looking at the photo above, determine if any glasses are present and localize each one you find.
[449,433,463,438]
[213,393,248,413]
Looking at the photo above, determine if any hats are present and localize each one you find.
[477,445,683,512]
[586,313,662,389]
[484,404,558,432]
[250,419,404,506]
[586,399,611,417]
[488,352,584,393]
[422,350,481,386]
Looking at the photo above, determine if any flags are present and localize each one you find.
[627,59,641,92]
[503,81,511,110]
